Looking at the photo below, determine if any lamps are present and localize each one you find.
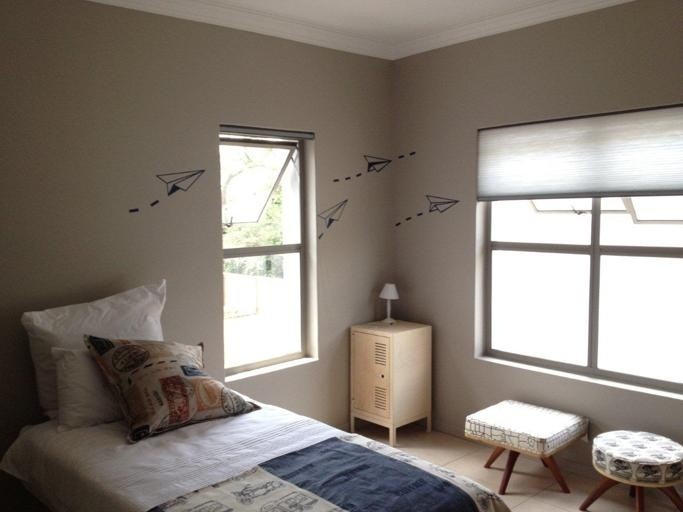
[378,283,399,324]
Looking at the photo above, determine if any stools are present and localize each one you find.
[464,399,589,495]
[581,428,683,511]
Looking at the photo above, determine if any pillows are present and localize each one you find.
[83,334,261,444]
[52,348,124,432]
[21,279,166,421]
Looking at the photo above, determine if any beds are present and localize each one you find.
[0,279,510,511]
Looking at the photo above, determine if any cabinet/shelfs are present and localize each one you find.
[349,319,432,447]
